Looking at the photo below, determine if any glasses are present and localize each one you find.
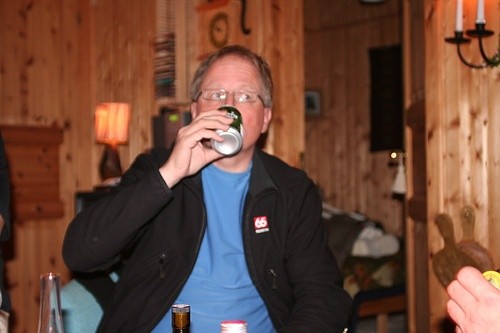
[195,88,266,109]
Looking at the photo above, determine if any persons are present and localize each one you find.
[447,266,500,333]
[62,45,344,333]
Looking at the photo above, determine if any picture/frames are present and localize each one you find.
[304,87,323,121]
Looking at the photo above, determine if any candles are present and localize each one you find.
[477,0,485,22]
[455,0,464,31]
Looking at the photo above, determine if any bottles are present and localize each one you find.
[171,304,190,333]
[38,273,65,333]
[221,320,248,333]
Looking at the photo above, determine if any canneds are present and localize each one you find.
[211,105,244,156]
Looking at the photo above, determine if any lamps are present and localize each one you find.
[94,101,132,181]
